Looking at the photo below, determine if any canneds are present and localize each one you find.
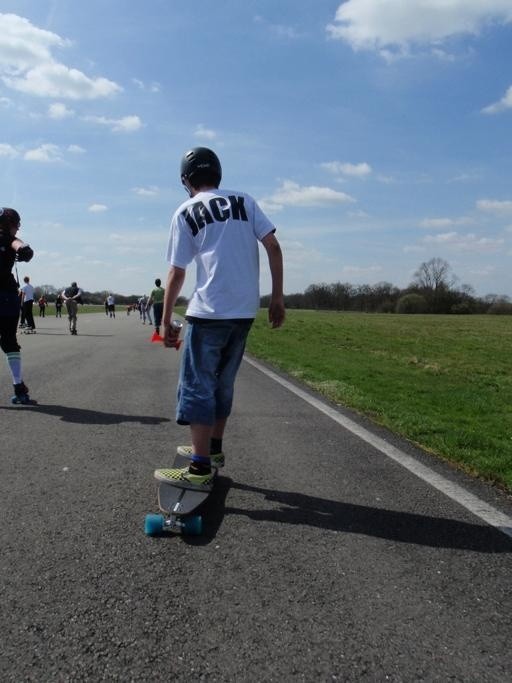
[164,320,183,348]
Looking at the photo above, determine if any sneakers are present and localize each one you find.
[177,445,225,468]
[155,466,215,493]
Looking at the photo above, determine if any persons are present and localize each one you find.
[1,208,34,400]
[152,146,284,486]
[17,276,164,334]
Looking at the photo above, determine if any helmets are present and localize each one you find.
[0,208,20,232]
[180,147,221,177]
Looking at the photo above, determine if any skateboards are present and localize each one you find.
[145,454,217,535]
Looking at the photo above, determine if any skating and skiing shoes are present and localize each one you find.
[11,382,30,404]
